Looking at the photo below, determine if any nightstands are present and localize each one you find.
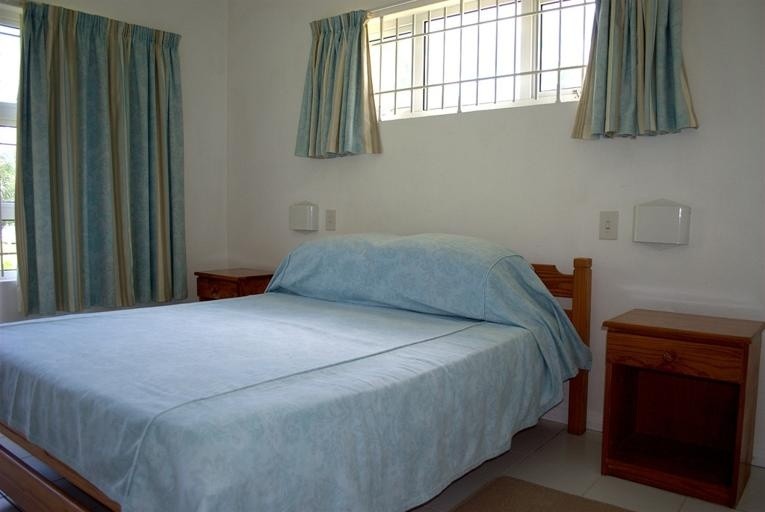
[601,309,765,509]
[193,269,274,302]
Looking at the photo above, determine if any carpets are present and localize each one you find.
[448,475,624,511]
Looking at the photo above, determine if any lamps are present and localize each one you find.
[632,200,691,247]
[288,203,317,231]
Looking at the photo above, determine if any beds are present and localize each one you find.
[0,257,593,512]
